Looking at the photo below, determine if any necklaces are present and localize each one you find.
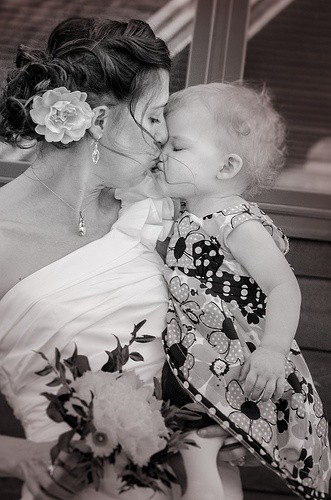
[29,163,102,237]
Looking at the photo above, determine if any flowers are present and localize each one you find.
[34,320,206,496]
[32,89,94,142]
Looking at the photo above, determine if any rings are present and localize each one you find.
[45,463,58,476]
[228,460,236,468]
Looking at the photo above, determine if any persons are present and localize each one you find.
[0,13,248,500]
[158,81,331,500]
